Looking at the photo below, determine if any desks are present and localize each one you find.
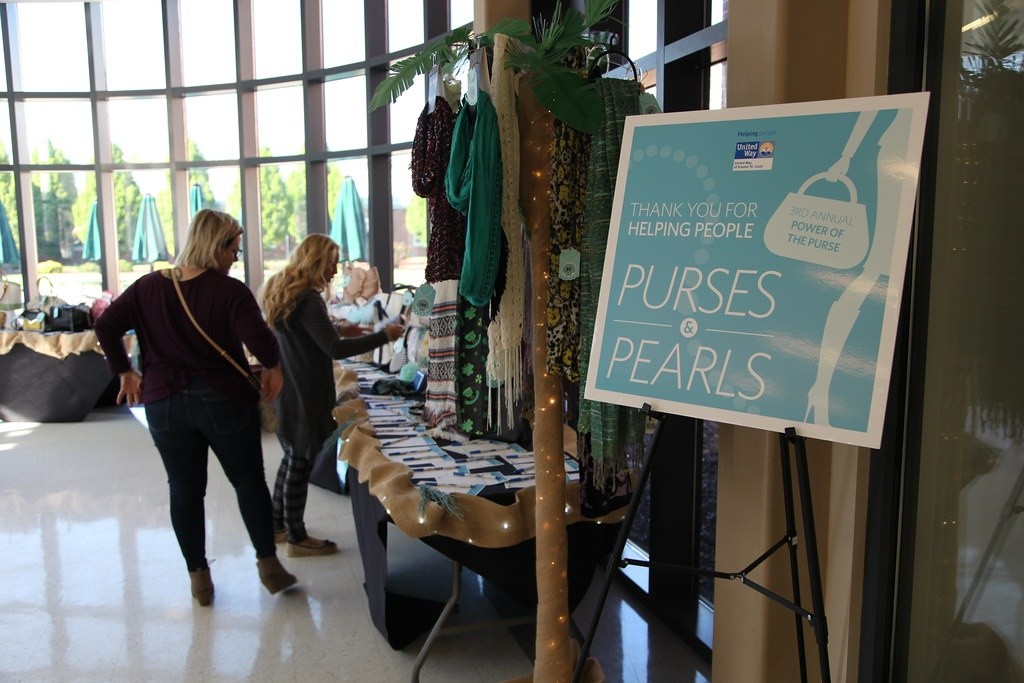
[333,360,640,683]
[1,329,130,423]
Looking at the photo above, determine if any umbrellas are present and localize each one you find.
[329,176,367,262]
[132,194,168,272]
[82,199,102,261]
[190,183,203,220]
[0,201,19,264]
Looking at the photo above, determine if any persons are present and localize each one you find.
[94,209,297,607]
[262,234,404,557]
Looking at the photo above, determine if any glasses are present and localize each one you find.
[226,247,243,258]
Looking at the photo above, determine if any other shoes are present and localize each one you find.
[275,529,289,543]
[286,537,337,558]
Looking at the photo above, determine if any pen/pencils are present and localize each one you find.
[360,383,373,385]
[451,440,492,446]
[375,401,406,406]
[506,454,533,458]
[454,472,501,479]
[402,434,426,437]
[513,465,534,474]
[364,396,392,400]
[381,419,406,422]
[508,476,534,482]
[455,457,496,463]
[412,466,461,472]
[404,455,448,460]
[389,449,431,456]
[470,446,510,454]
[425,482,475,490]
[383,437,409,446]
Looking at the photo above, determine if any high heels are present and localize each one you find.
[189,571,214,606]
[257,557,298,594]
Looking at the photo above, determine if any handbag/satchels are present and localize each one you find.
[257,386,278,434]
[386,283,419,321]
[0,270,112,335]
[373,300,395,367]
